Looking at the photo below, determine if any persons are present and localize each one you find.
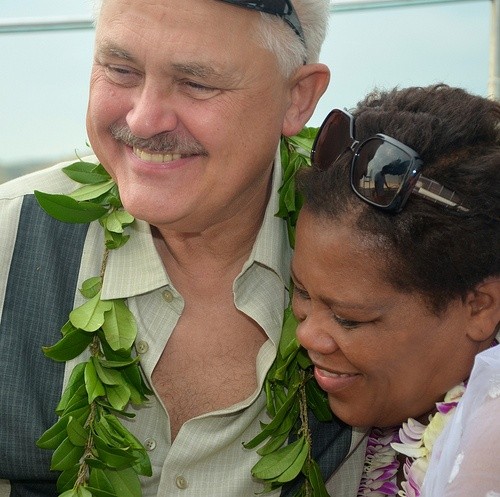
[291,82,500,497]
[0,0,370,497]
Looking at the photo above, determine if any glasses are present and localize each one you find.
[218,0,310,69]
[308,106,498,224]
